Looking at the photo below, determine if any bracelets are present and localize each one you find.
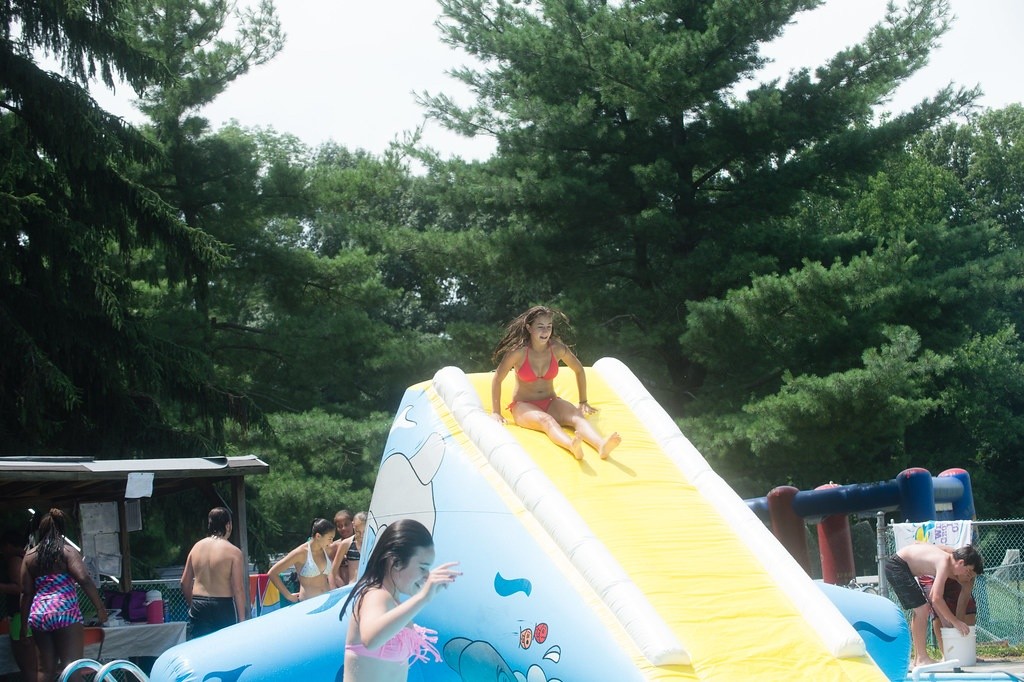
[97,608,103,613]
[579,400,588,404]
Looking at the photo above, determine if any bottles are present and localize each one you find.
[164,601,170,623]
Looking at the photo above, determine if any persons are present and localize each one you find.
[330,512,368,590]
[326,510,357,586]
[338,519,464,682]
[0,529,43,682]
[180,508,247,642]
[917,543,985,656]
[19,512,109,682]
[885,543,981,668]
[266,518,336,603]
[490,307,621,461]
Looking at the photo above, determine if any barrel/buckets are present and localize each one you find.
[940,626,978,667]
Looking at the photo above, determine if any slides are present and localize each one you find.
[146,356,911,680]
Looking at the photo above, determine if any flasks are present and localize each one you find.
[143,589,164,624]
